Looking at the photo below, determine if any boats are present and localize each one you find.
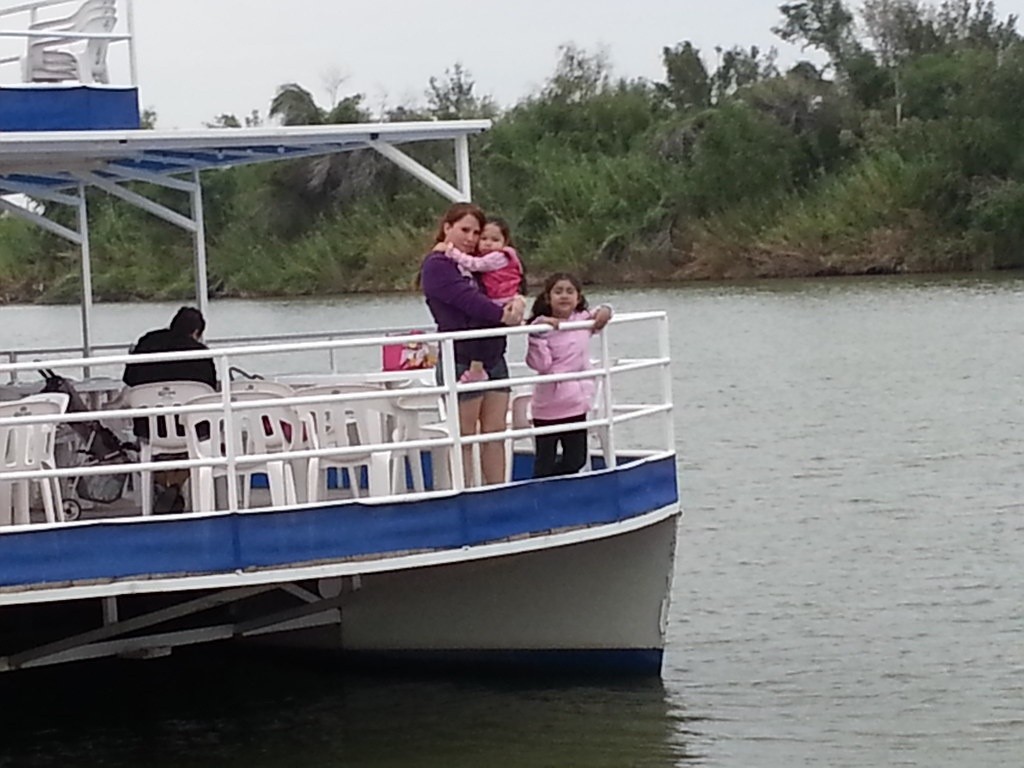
[0,0,682,680]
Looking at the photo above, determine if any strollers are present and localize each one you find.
[33,359,163,524]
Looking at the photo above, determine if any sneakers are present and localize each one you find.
[458,369,488,383]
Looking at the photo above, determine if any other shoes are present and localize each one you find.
[153,483,185,515]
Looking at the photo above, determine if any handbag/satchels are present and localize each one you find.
[383,331,439,372]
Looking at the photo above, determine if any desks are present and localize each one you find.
[274,370,437,499]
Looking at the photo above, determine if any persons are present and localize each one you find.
[415,203,525,488]
[526,272,615,480]
[432,216,526,384]
[122,307,217,514]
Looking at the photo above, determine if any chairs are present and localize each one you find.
[24,0,118,85]
[0,356,618,525]
